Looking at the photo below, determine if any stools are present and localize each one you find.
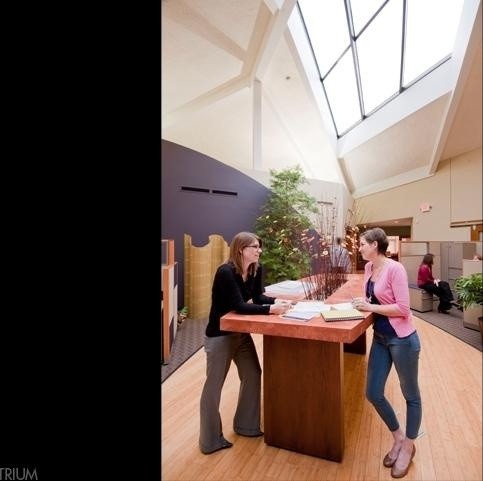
[407,283,432,313]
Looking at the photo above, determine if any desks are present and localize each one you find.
[220,272,375,464]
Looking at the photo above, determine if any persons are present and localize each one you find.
[198,231,294,455]
[330,237,351,274]
[350,228,421,479]
[416,253,460,316]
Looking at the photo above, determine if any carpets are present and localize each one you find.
[160,313,204,387]
[411,294,482,355]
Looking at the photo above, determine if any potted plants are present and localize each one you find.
[453,271,483,331]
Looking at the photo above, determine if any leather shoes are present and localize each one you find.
[390,443,417,477]
[381,444,400,467]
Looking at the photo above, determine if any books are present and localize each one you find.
[321,309,365,322]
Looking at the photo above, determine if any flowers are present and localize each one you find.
[258,195,376,300]
